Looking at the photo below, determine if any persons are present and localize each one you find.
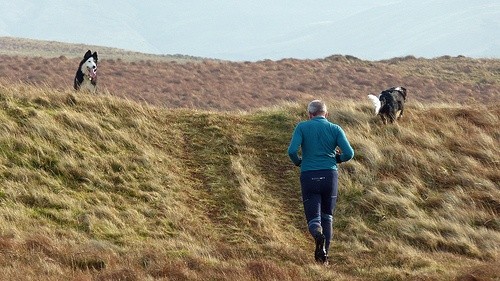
[288,100,354,264]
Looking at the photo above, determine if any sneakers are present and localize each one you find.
[323,260,329,266]
[314,233,327,266]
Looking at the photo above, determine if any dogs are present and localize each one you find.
[74,49,98,94]
[367,87,406,125]
